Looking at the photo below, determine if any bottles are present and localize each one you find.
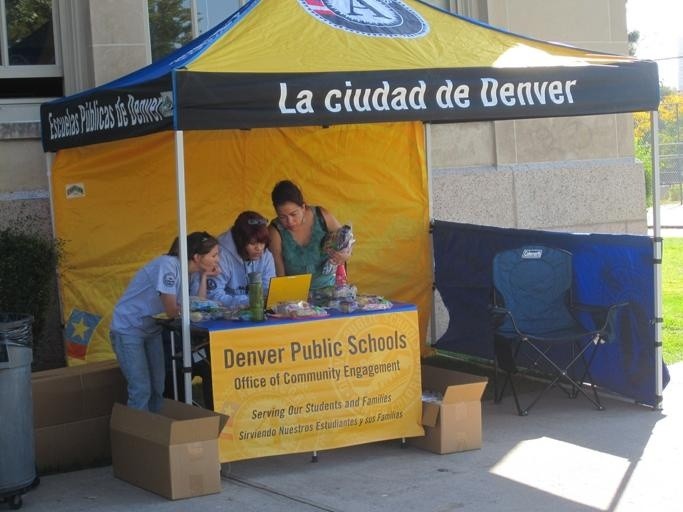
[247,272,264,309]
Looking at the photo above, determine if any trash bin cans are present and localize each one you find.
[0,338,41,511]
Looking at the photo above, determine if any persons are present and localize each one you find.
[162,212,276,410]
[109,231,222,413]
[269,179,352,294]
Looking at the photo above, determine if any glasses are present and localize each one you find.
[247,219,269,225]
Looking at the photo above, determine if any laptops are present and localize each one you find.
[263,272,313,311]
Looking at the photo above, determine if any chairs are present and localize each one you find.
[484,245,632,418]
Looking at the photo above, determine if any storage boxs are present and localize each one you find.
[414,360,491,456]
[107,395,231,505]
[33,414,110,478]
[29,356,128,427]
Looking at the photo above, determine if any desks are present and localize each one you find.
[155,298,425,475]
